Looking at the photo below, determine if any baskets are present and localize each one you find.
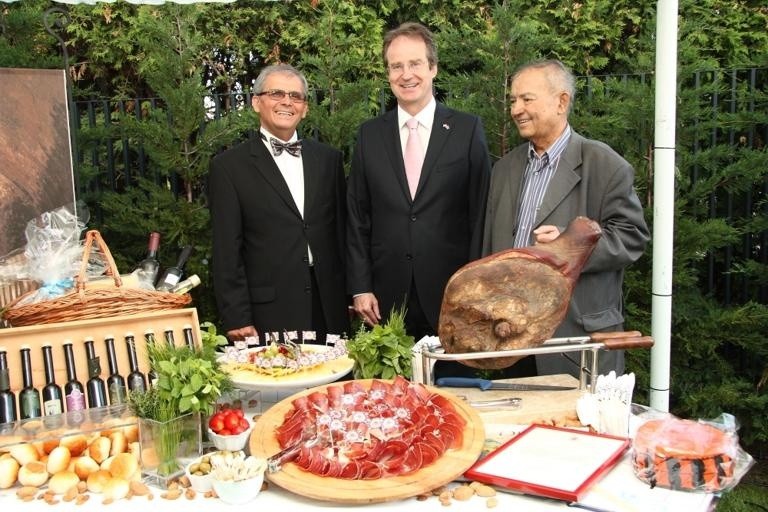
[2,231,192,326]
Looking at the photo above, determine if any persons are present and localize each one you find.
[481,61,652,387]
[207,63,357,352]
[344,21,497,378]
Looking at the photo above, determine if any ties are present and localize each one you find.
[403,120,425,201]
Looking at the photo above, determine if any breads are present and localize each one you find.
[0,418,159,500]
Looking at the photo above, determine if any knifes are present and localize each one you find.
[437,376,578,392]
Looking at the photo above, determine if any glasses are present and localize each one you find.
[258,89,306,103]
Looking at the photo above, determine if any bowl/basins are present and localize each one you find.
[216,343,356,406]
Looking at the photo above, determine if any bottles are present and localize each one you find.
[62,344,85,429]
[41,345,65,432]
[156,243,194,292]
[124,326,196,393]
[84,340,108,424]
[136,231,161,290]
[104,338,127,418]
[0,351,17,436]
[18,348,42,434]
[168,274,202,299]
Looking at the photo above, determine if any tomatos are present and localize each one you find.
[210,408,249,435]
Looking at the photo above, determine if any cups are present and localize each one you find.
[184,416,268,507]
[140,408,203,488]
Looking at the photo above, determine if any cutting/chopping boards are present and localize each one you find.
[247,379,487,506]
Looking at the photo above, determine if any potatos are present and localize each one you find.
[189,451,240,476]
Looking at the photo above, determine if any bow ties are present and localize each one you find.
[269,137,302,157]
